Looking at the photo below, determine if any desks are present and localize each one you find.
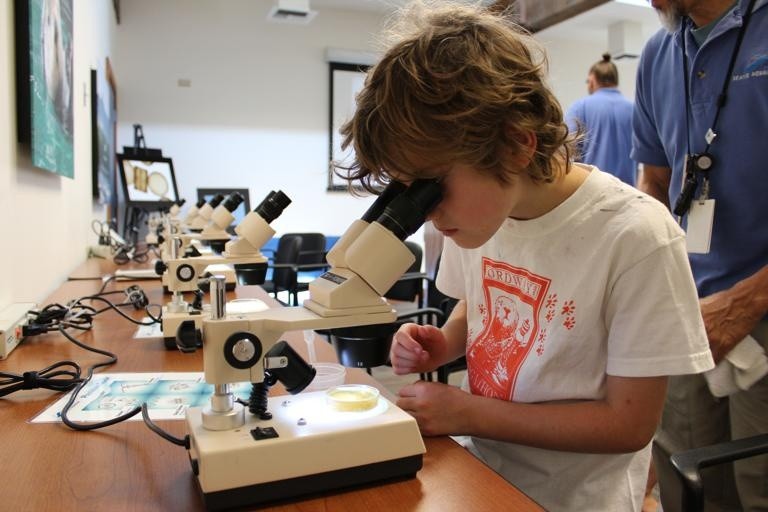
[68,241,160,280]
[0,280,551,512]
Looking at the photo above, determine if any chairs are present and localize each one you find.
[262,233,466,383]
[670,433,768,512]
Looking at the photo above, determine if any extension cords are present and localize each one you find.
[0,302,39,361]
[91,236,118,258]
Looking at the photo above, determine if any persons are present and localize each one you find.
[558,52,638,187]
[326,0,715,512]
[631,0,768,511]
